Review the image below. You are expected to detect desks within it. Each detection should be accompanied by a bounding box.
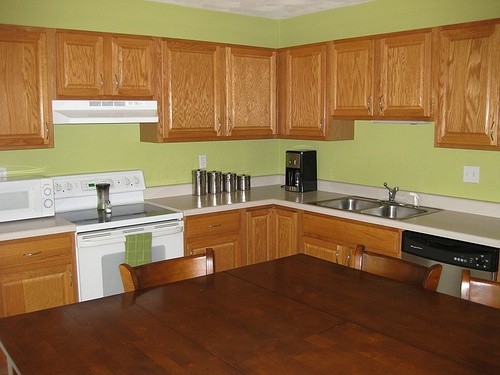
[1,253,500,375]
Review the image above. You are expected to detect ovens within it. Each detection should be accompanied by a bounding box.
[75,220,185,303]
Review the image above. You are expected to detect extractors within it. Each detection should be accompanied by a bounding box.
[51,99,159,124]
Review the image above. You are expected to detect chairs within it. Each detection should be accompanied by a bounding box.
[119,248,217,292]
[459,269,499,308]
[353,243,442,291]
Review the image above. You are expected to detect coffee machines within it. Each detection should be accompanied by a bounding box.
[285,149,317,192]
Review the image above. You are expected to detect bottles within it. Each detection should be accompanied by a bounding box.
[96,183,111,210]
[105,200,112,213]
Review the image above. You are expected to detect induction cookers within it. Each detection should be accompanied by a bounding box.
[49,170,184,233]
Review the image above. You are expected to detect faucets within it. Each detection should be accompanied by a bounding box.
[383,182,406,206]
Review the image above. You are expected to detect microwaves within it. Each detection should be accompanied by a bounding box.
[0,174,56,223]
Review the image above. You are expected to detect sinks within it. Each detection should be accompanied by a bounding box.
[304,196,381,210]
[360,206,441,220]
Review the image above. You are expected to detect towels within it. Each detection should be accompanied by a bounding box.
[124,232,152,267]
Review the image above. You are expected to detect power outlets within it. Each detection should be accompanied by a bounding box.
[463,165,480,183]
[0,168,7,179]
[199,155,206,169]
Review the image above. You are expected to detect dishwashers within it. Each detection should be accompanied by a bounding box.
[400,229,500,300]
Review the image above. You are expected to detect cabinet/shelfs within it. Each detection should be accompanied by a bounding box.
[277,43,354,141]
[184,210,243,273]
[1,22,57,151]
[54,30,154,101]
[296,212,403,273]
[330,29,435,122]
[433,17,500,150]
[141,40,278,143]
[0,233,78,317]
[244,206,274,265]
[275,204,298,259]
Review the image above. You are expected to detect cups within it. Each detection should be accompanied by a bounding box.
[208,170,222,194]
[238,174,250,190]
[222,172,237,192]
[192,169,206,196]
